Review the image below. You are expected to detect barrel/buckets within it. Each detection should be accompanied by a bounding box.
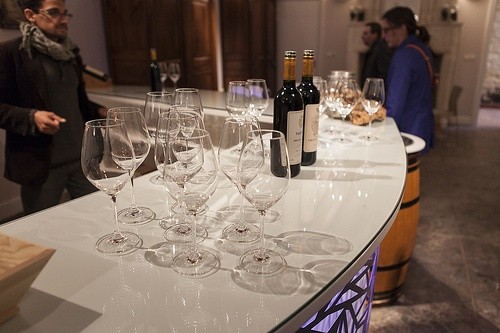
[365,151,424,298]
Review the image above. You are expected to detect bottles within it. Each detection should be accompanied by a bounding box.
[149,47,162,92]
[270,50,304,177]
[82,65,109,82]
[297,49,320,167]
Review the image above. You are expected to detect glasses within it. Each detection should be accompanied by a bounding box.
[383,24,401,34]
[30,7,73,20]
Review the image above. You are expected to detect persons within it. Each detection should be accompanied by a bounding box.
[379,6,435,157]
[360,22,396,92]
[0,0,109,215]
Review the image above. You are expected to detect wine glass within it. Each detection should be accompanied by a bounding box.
[313,71,360,148]
[158,62,168,91]
[360,77,385,142]
[80,78,291,278]
[168,63,181,90]
